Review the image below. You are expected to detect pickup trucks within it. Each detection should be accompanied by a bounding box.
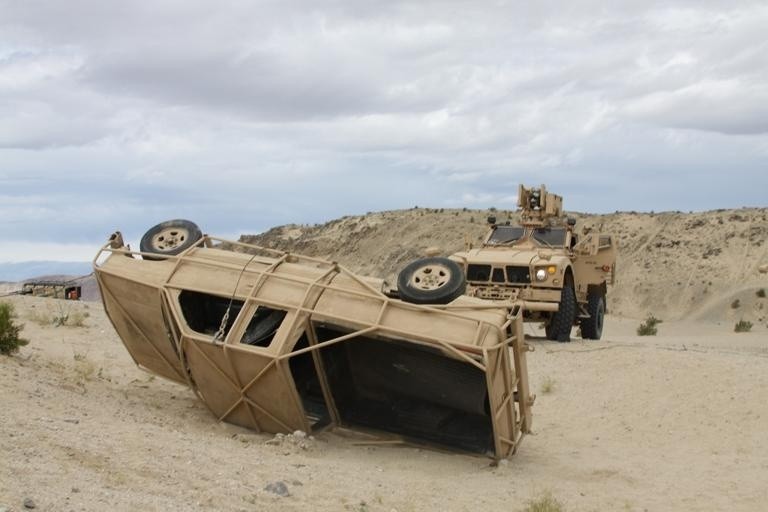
[91,216,537,469]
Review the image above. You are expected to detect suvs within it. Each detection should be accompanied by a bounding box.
[12,281,82,300]
[426,184,620,342]
